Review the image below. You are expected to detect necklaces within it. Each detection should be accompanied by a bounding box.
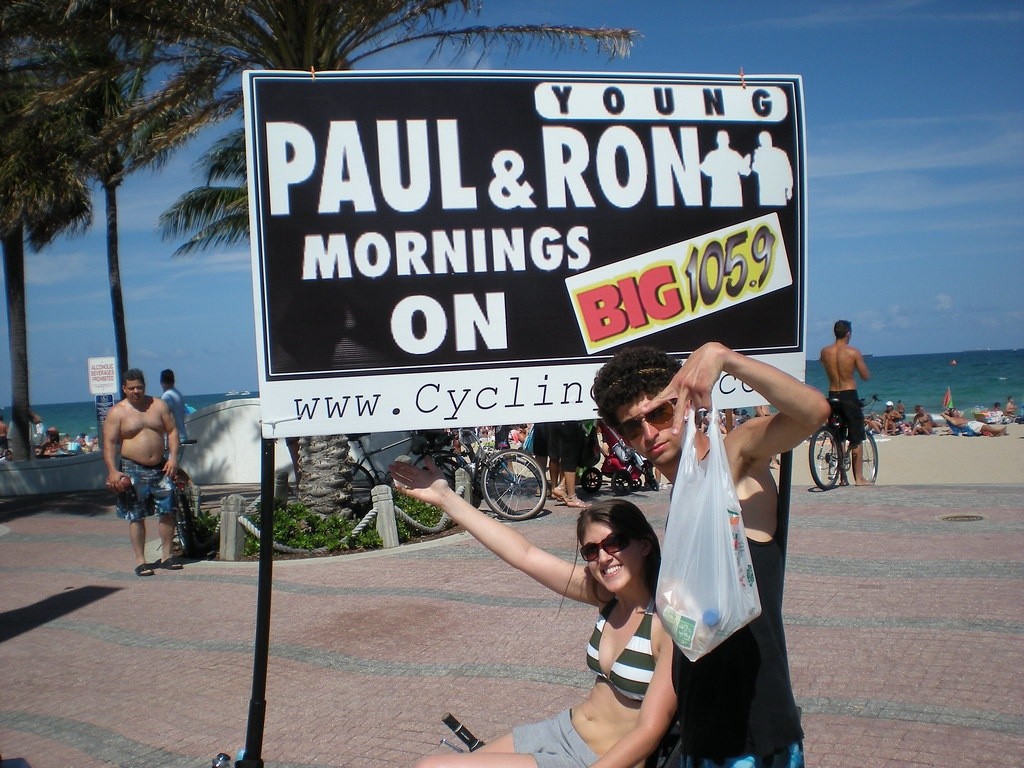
[702,449,710,459]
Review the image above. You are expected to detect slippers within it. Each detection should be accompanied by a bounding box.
[134,563,154,576]
[157,558,183,570]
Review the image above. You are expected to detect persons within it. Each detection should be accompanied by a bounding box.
[103,369,183,576]
[991,397,1017,423]
[479,420,602,508]
[755,406,779,469]
[388,456,678,768]
[911,405,941,435]
[0,416,8,455]
[697,405,751,433]
[863,400,904,436]
[160,369,187,443]
[593,341,832,768]
[941,408,1008,437]
[655,467,668,489]
[7,408,98,458]
[821,320,873,487]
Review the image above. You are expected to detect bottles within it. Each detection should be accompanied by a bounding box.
[470,457,476,479]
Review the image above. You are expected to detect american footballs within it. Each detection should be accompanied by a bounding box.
[106,476,132,492]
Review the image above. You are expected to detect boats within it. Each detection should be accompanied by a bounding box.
[0,398,439,497]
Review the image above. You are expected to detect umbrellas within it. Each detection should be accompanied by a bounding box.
[944,387,953,409]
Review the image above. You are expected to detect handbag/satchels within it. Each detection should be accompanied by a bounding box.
[655,401,762,663]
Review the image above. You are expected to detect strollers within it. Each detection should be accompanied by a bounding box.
[582,419,660,496]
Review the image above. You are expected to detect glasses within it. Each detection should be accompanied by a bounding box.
[615,399,677,441]
[579,531,630,561]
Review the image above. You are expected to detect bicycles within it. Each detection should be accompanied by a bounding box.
[342,428,548,522]
[808,392,879,491]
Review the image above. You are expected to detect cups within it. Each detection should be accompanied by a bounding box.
[107,476,131,492]
